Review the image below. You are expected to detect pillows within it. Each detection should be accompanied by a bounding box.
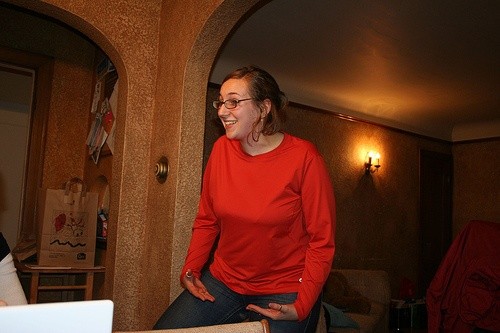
[325,305,358,328]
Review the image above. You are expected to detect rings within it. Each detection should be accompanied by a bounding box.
[279,306,282,311]
[185,272,191,278]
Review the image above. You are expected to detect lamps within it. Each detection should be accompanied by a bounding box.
[364,150,381,176]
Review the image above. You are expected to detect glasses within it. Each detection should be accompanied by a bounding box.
[212,96,256,110]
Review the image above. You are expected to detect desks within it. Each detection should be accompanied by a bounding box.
[20,263,106,304]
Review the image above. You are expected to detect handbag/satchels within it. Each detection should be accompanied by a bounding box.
[37,179,99,268]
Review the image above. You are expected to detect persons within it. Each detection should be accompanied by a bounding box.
[0,232,28,306]
[153,65,336,333]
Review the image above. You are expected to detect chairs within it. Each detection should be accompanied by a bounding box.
[320,267,391,333]
